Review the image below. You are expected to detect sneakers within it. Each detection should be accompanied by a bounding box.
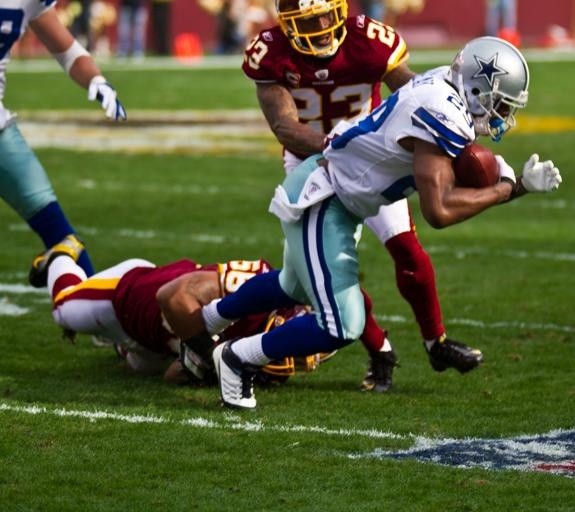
[423,334,483,374]
[361,351,397,394]
[160,310,209,386]
[210,338,262,411]
[28,233,86,291]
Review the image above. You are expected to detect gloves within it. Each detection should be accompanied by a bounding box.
[87,75,126,122]
[494,153,517,198]
[521,153,562,194]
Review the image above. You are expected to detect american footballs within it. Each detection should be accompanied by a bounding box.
[454,145,500,188]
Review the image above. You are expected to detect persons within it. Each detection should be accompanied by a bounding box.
[241,2,485,395]
[65,1,172,58]
[200,34,567,413]
[27,220,372,390]
[360,1,384,22]
[487,0,517,40]
[0,1,130,284]
[218,0,248,55]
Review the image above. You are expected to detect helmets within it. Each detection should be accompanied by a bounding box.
[444,35,531,114]
[274,0,348,60]
[274,303,338,369]
